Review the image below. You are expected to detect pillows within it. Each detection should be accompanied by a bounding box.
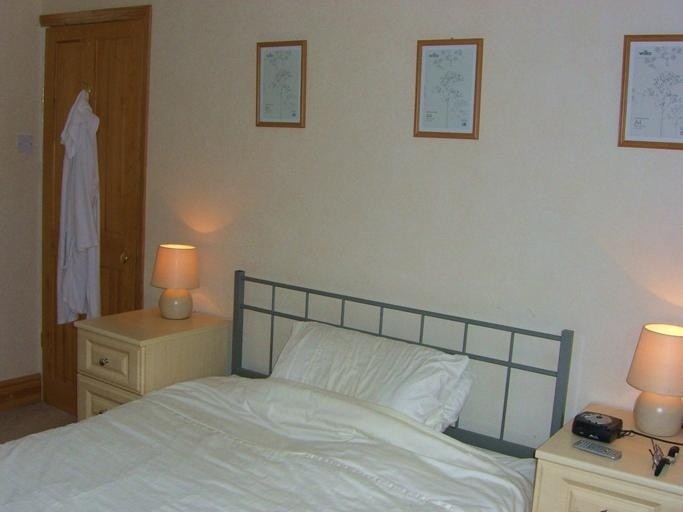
[270,319,469,425]
[425,375,473,433]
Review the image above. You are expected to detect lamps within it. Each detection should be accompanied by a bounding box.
[626,323,683,437]
[150,243,199,320]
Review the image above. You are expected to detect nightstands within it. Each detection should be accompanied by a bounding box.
[531,402,683,512]
[73,308,233,421]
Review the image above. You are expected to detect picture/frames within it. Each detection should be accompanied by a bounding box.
[618,34,683,150]
[255,40,308,129]
[413,37,484,141]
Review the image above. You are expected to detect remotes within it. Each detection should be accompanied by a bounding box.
[573,439,622,461]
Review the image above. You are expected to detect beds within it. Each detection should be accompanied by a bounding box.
[0,270,575,512]
